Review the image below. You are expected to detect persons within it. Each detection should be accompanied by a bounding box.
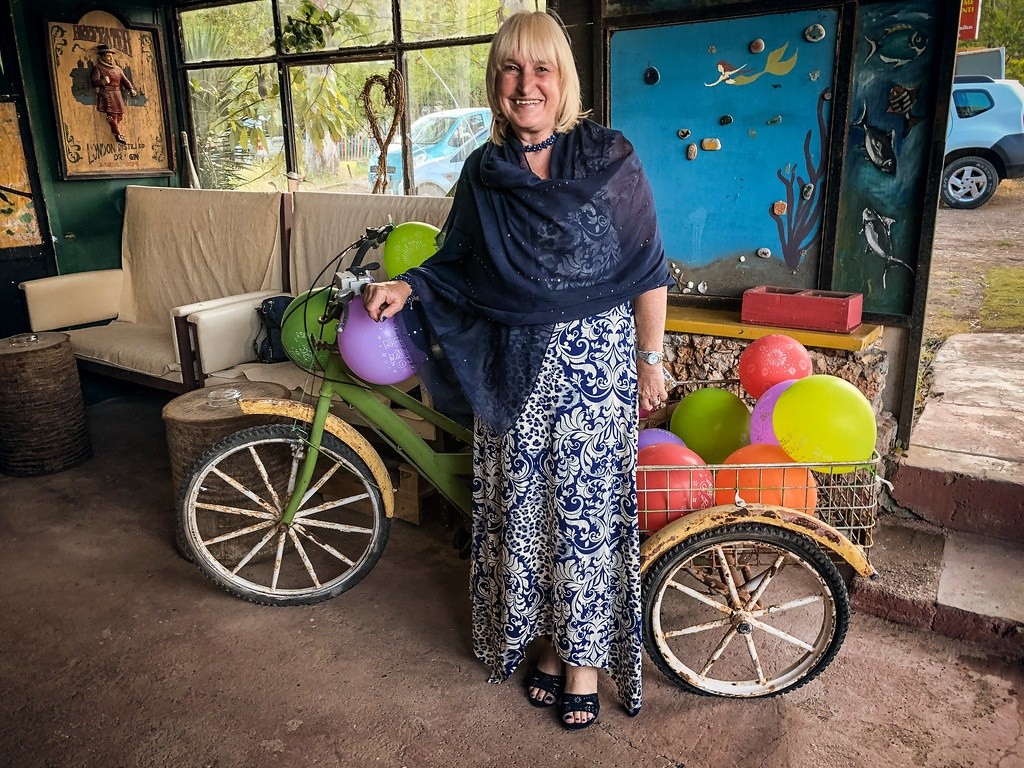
[92,45,137,144]
[361,12,677,730]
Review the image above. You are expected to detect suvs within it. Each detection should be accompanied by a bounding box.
[940,75,1024,210]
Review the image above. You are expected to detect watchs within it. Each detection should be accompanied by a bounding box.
[637,350,664,365]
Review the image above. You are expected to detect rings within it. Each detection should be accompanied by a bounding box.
[651,399,658,404]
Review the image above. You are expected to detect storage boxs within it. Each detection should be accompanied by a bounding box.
[742,284,863,332]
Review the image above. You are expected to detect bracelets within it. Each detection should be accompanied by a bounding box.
[391,275,417,304]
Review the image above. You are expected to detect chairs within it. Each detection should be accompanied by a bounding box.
[17,184,455,454]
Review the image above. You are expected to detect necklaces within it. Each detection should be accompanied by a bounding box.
[524,133,559,152]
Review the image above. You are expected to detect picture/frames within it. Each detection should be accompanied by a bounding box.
[0,95,53,259]
[593,0,860,310]
[44,20,180,181]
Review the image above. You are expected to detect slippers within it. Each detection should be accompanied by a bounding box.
[561,692,600,729]
[527,655,563,707]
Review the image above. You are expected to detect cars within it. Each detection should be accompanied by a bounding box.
[227,113,287,165]
[366,107,493,197]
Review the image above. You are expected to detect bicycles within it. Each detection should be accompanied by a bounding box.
[175,213,882,701]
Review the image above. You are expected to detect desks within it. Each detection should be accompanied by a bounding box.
[0,334,93,474]
[163,379,315,565]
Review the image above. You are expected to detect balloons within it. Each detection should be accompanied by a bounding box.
[638,427,686,449]
[636,443,715,535]
[749,379,796,445]
[715,445,816,516]
[670,388,752,475]
[280,287,343,372]
[384,222,447,281]
[773,374,876,474]
[337,295,423,385]
[739,334,813,401]
